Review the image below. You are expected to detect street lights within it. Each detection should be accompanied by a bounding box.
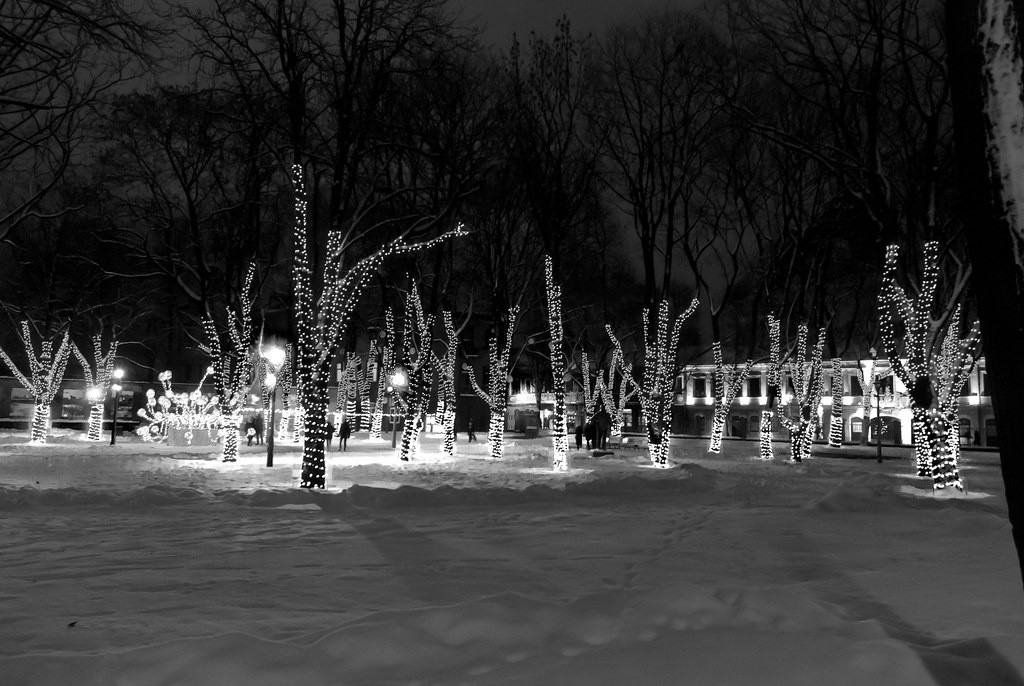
[391,373,405,450]
[872,380,883,464]
[109,369,125,446]
[266,349,286,469]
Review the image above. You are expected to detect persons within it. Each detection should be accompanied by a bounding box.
[338,419,350,452]
[324,422,336,451]
[574,423,591,450]
[245,413,264,445]
[467,417,477,442]
[590,404,613,449]
